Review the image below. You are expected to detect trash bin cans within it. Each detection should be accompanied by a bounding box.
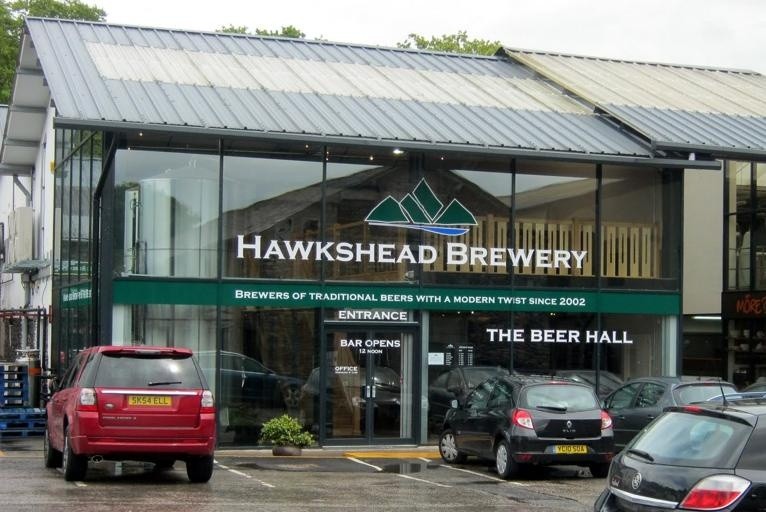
[16,349,41,408]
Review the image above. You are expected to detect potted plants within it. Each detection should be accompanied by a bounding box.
[256,411,315,457]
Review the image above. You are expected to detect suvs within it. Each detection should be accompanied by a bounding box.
[41,345,216,483]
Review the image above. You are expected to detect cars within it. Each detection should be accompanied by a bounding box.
[427,366,625,442]
[193,350,306,410]
[591,397,765,511]
[438,375,616,479]
[602,374,738,446]
[297,365,402,439]
